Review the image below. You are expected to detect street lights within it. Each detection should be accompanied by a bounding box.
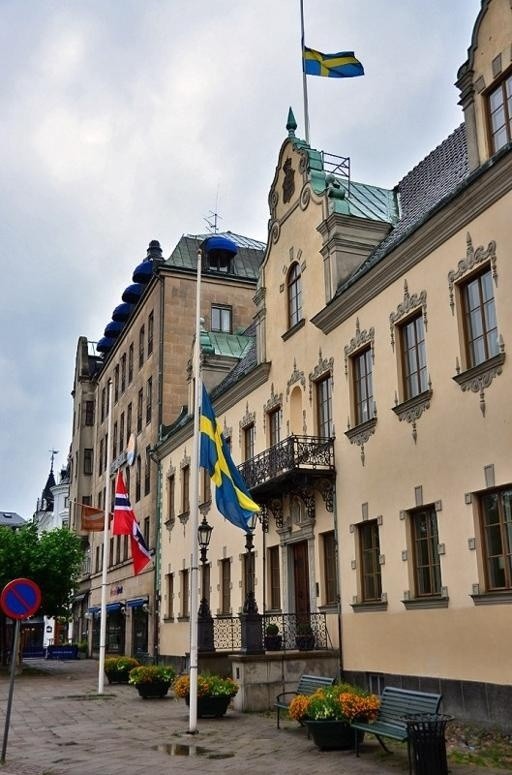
[198,515,215,653]
[238,513,266,655]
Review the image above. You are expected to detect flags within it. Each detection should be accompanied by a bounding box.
[301,45,366,78]
[81,506,114,532]
[113,470,152,577]
[197,382,261,534]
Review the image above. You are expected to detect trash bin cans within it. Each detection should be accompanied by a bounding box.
[400,712,455,775]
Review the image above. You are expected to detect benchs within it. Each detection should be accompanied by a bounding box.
[349,686,444,775]
[272,674,336,739]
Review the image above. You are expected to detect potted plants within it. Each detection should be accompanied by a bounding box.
[288,682,380,750]
[97,653,140,685]
[172,672,240,717]
[128,664,176,697]
